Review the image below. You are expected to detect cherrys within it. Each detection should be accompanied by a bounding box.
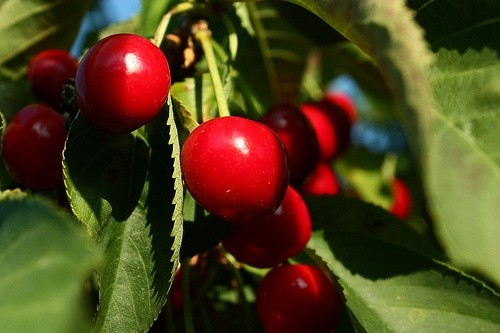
[4,33,412,332]
